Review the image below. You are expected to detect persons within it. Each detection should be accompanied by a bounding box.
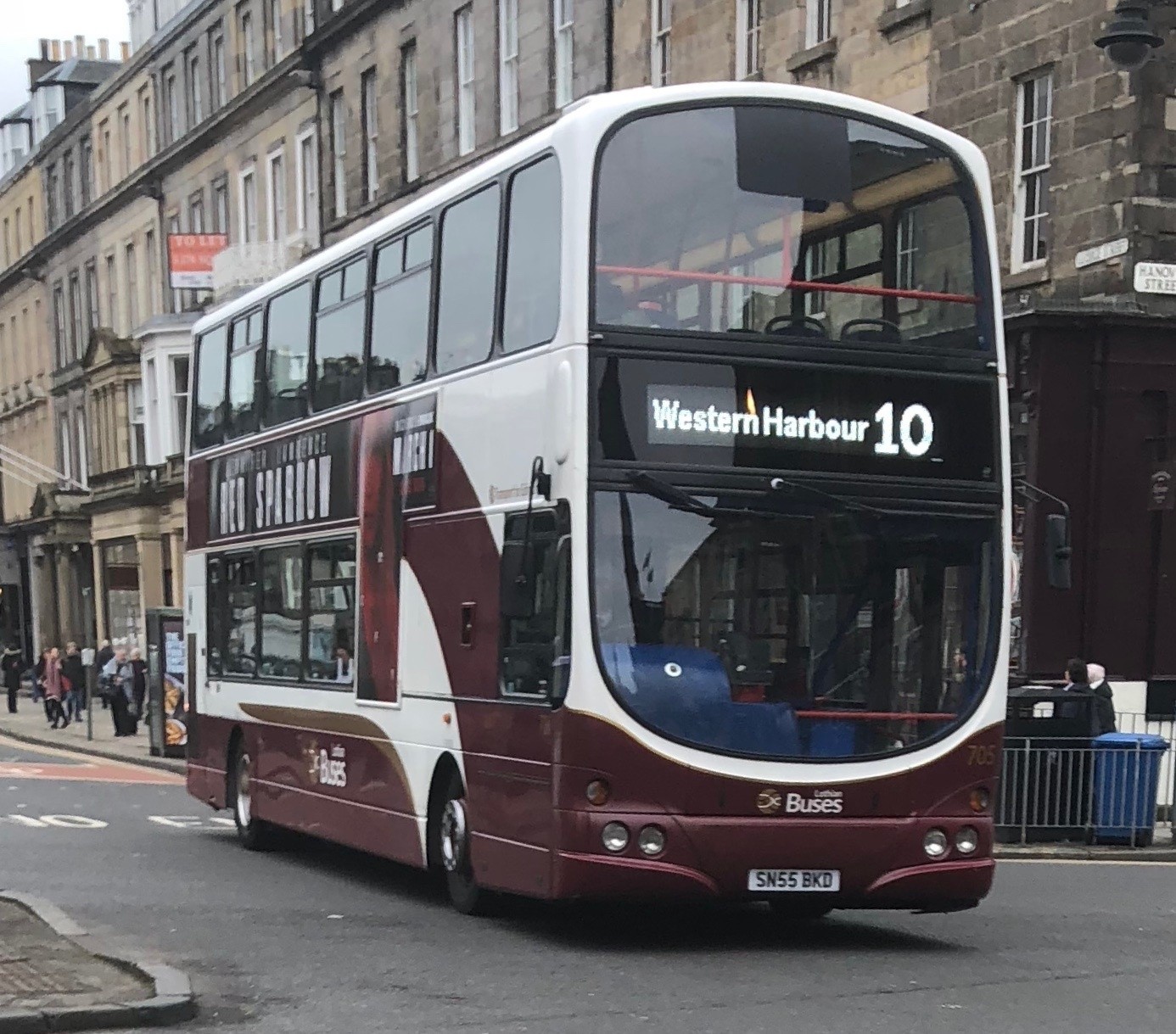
[1,639,147,737]
[954,641,967,681]
[1053,658,1117,735]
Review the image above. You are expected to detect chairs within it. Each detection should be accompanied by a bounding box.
[218,649,337,682]
[192,358,394,443]
[760,314,901,342]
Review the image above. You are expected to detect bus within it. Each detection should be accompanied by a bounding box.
[186,80,1074,931]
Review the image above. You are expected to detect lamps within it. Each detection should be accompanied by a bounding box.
[286,67,325,91]
[22,267,46,286]
[135,181,163,200]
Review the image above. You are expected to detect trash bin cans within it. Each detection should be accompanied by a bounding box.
[1092,732,1167,848]
[996,684,1096,846]
